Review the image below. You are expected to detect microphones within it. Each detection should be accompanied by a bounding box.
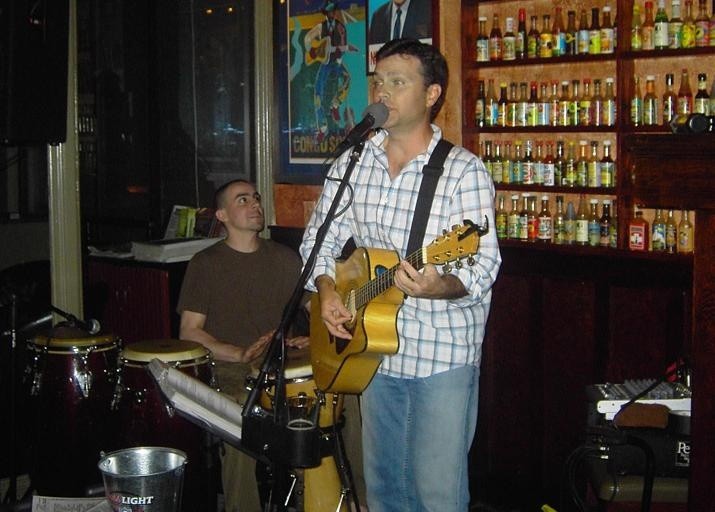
[41,302,101,335]
[331,103,389,158]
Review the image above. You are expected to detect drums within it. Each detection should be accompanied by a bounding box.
[22,324,122,497]
[250,342,348,512]
[114,338,223,511]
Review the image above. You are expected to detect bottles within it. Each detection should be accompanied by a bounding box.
[569,80,581,125]
[474,82,486,126]
[587,141,600,188]
[502,17,513,60]
[513,140,522,185]
[515,9,527,60]
[600,200,610,247]
[580,11,590,54]
[489,12,500,60]
[539,16,551,57]
[667,113,710,133]
[554,196,565,244]
[554,138,567,187]
[581,80,592,125]
[669,2,682,48]
[533,141,544,185]
[678,66,693,114]
[520,193,529,241]
[710,1,715,47]
[576,194,588,246]
[663,72,677,124]
[548,81,559,125]
[507,83,516,125]
[629,211,649,251]
[503,142,513,184]
[528,196,538,242]
[602,79,617,125]
[522,140,533,185]
[477,17,487,62]
[559,82,570,125]
[631,5,641,50]
[630,75,643,124]
[486,79,499,125]
[538,196,551,243]
[666,210,678,255]
[610,199,617,247]
[538,81,550,125]
[477,140,483,162]
[695,0,709,45]
[679,210,693,254]
[483,141,492,176]
[525,15,539,58]
[681,1,695,47]
[551,9,567,53]
[493,142,503,183]
[655,1,668,49]
[600,142,613,187]
[593,78,602,126]
[576,140,588,186]
[653,209,665,252]
[641,2,655,49]
[496,194,508,238]
[614,7,618,50]
[566,140,576,187]
[600,7,613,52]
[567,12,578,54]
[508,195,520,239]
[527,82,539,125]
[517,82,528,126]
[588,199,600,246]
[543,140,555,186]
[565,200,576,245]
[694,72,710,115]
[498,84,508,125]
[588,8,600,52]
[644,74,658,125]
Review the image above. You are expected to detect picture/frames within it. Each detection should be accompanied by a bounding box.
[272,0,368,184]
[366,0,440,75]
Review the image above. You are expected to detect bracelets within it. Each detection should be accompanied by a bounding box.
[240,348,248,365]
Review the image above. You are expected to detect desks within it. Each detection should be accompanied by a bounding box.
[82,238,205,341]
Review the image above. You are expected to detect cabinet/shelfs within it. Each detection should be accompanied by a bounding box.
[460,1,715,457]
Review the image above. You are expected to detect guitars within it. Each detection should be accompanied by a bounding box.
[310,215,488,393]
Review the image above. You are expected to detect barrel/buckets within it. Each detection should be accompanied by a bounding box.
[97,446,190,512]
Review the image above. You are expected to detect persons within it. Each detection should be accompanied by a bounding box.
[175,180,310,512]
[370,0,433,42]
[298,37,502,512]
